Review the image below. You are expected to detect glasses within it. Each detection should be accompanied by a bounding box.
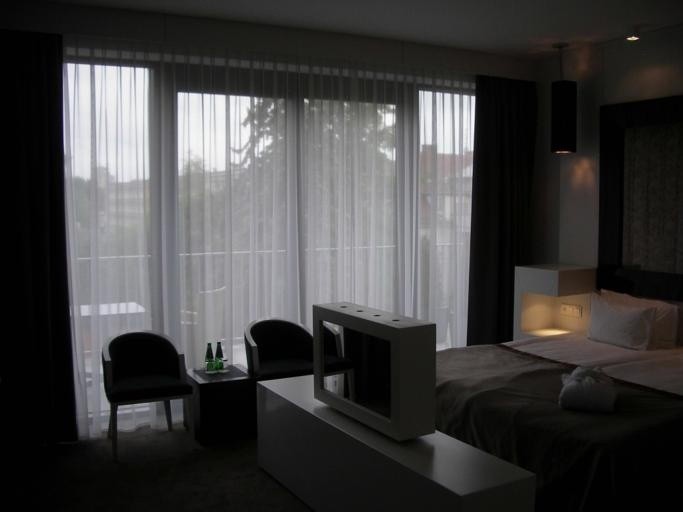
[547,40,580,156]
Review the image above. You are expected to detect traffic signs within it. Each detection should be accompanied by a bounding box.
[558,303,582,318]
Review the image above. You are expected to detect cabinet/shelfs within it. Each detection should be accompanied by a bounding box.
[215,341,224,370]
[204,342,215,372]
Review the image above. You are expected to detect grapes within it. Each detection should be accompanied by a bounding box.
[511,262,596,343]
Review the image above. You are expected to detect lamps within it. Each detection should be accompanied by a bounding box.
[244,318,314,379]
[99,331,194,440]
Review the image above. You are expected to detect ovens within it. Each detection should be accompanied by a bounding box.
[433,272,683,511]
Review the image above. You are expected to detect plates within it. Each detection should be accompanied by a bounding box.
[256,375,536,511]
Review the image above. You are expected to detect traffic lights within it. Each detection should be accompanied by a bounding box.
[588,291,654,352]
[632,296,680,349]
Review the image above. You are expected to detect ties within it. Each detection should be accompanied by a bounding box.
[311,302,438,444]
